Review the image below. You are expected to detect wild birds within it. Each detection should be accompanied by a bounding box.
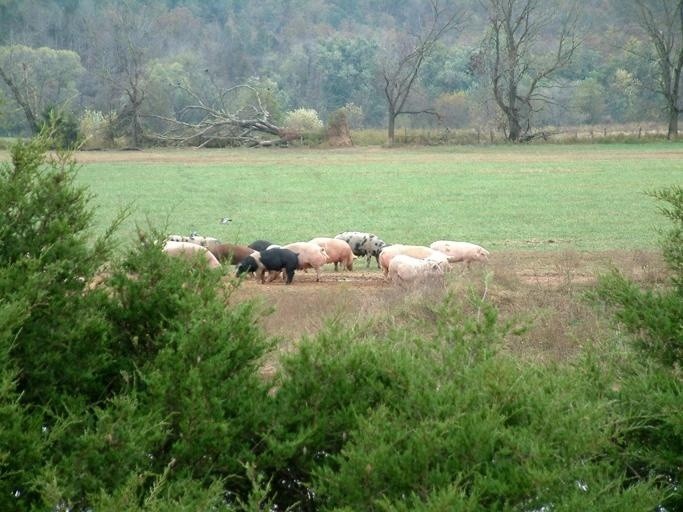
[220,217,232,224]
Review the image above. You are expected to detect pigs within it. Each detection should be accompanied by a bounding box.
[150,232,392,286]
[378,239,487,285]
